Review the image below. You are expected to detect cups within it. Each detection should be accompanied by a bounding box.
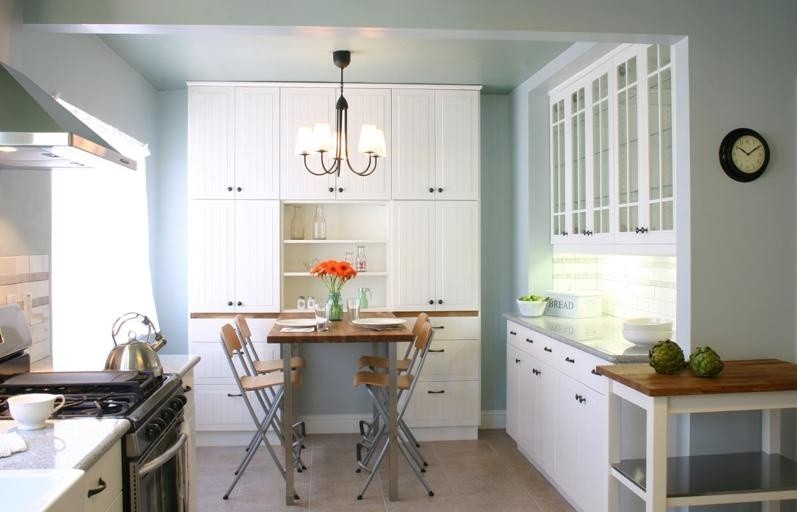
[6,393,65,430]
[346,298,361,322]
[315,304,329,332]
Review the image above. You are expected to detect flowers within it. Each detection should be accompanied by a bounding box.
[309,259,358,312]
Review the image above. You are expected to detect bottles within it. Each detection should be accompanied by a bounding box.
[290,206,305,240]
[356,245,367,272]
[312,204,326,240]
[345,252,354,266]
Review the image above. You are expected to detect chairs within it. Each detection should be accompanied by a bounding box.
[233,314,307,470]
[220,324,302,500]
[354,322,434,500]
[357,312,430,466]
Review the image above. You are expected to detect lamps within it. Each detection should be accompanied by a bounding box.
[296,50,387,176]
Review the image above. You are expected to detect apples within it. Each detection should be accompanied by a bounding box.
[519,294,544,302]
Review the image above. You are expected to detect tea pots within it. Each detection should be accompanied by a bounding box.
[104,312,167,381]
[276,318,327,328]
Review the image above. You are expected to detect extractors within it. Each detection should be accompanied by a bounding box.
[1,60,136,172]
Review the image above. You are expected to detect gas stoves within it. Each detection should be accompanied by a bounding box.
[0,373,194,458]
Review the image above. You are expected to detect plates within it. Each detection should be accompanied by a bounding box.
[352,318,406,330]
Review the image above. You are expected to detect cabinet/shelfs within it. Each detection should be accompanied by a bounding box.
[82,439,123,512]
[186,81,280,447]
[596,359,797,512]
[548,43,679,256]
[181,368,195,512]
[517,327,557,488]
[280,83,391,434]
[557,335,621,512]
[506,317,522,443]
[391,84,481,440]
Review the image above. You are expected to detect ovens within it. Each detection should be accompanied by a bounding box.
[122,410,188,512]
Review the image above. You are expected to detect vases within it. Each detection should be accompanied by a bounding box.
[326,292,344,321]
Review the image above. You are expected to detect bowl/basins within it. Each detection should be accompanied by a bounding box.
[517,299,548,318]
[624,318,673,331]
[623,331,673,347]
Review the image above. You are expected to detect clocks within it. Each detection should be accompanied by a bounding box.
[718,128,770,183]
